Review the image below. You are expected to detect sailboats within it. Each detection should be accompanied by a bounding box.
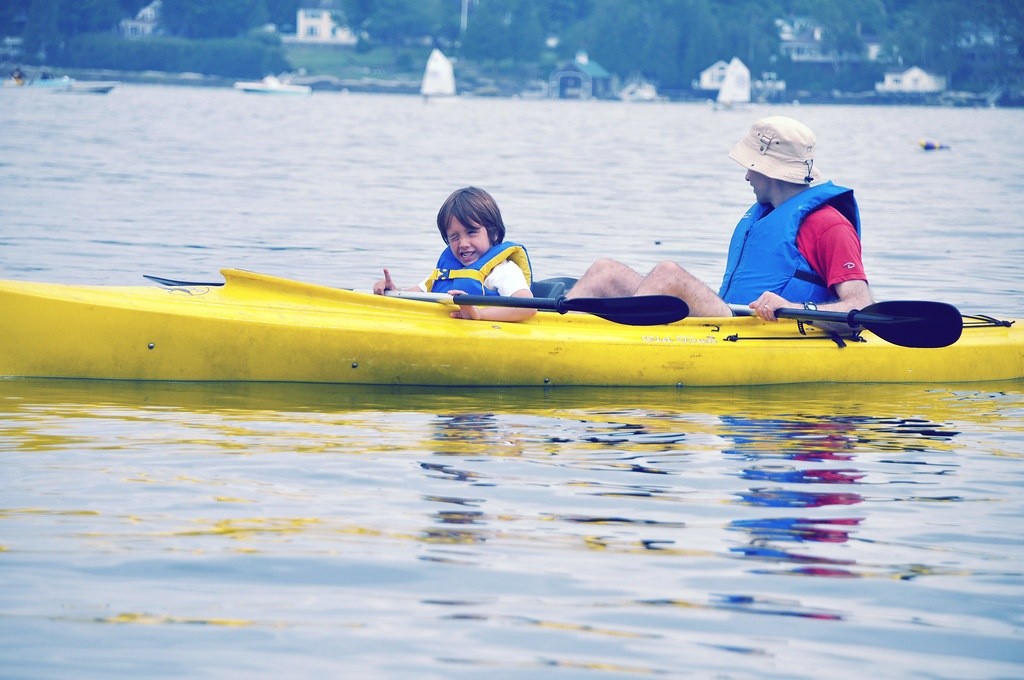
[417,47,459,100]
[712,53,752,112]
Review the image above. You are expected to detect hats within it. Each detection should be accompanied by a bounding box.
[729,116,827,184]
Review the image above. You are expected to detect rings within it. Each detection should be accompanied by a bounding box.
[765,305,771,311]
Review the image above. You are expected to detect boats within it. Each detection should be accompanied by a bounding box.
[1,266,1024,389]
[232,73,312,96]
[1,71,122,111]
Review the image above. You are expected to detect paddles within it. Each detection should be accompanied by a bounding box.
[141,272,690,327]
[535,276,963,348]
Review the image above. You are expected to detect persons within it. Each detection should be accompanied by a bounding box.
[374,187,537,321]
[566,115,876,337]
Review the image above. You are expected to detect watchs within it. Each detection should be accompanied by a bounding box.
[797,300,818,336]
